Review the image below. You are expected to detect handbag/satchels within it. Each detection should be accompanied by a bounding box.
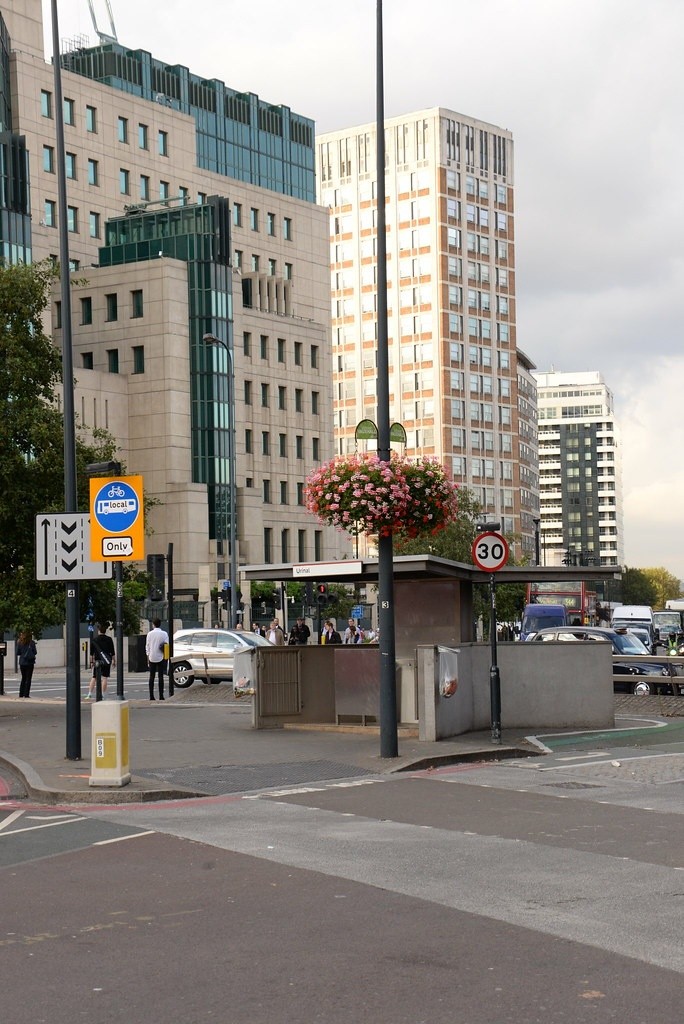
[23,644,36,662]
[99,650,112,665]
[159,641,167,652]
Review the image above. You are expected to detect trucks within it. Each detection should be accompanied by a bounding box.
[653,610,684,639]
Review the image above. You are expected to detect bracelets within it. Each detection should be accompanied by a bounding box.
[90,662,93,663]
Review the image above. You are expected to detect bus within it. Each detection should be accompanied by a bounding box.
[520,603,571,642]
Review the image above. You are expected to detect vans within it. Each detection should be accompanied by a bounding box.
[611,604,655,632]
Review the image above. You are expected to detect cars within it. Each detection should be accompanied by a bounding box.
[164,627,279,687]
[532,626,681,696]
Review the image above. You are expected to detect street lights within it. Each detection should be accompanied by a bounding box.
[202,332,238,629]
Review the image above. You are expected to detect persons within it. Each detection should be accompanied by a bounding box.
[84,624,117,700]
[16,629,37,698]
[214,616,380,644]
[145,619,169,700]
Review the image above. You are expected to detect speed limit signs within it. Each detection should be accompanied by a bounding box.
[472,531,509,572]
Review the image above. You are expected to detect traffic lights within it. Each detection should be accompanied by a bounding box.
[317,584,339,606]
